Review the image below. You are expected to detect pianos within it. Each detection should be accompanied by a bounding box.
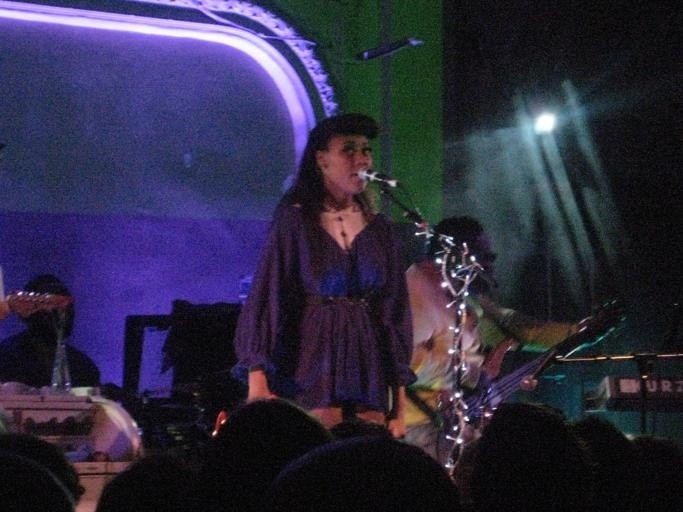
[1,380,101,432]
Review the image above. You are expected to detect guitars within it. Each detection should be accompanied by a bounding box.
[2,290,71,317]
[388,300,627,466]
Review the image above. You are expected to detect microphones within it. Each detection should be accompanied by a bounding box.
[356,167,401,189]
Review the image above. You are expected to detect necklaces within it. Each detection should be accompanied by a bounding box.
[335,208,351,249]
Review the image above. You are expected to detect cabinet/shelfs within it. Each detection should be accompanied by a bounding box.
[0,380,144,512]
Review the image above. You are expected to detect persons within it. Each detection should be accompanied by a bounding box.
[389,211,512,428]
[0,395,683,510]
[231,111,420,439]
[0,273,102,388]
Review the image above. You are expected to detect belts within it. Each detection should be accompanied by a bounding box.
[295,293,377,311]
